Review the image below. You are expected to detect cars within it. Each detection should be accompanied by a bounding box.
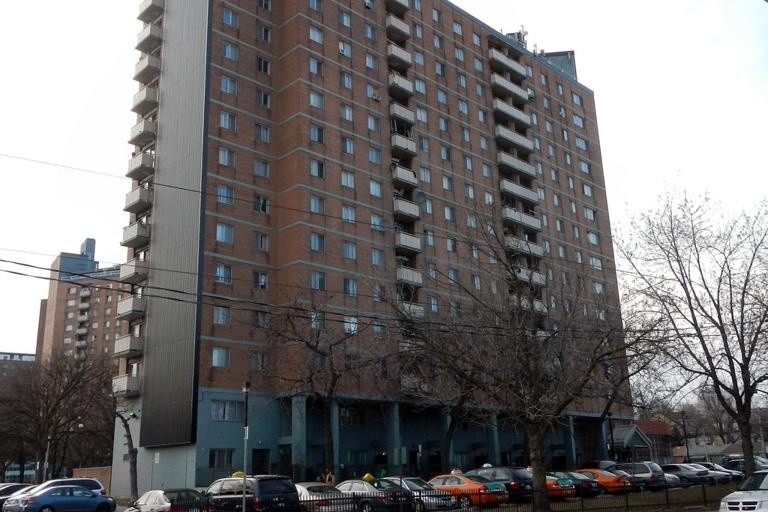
[0,475,116,510]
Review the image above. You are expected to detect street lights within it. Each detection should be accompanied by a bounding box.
[606,412,617,462]
[679,410,690,462]
[238,382,250,510]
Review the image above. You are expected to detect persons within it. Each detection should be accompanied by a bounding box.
[324,469,336,486]
